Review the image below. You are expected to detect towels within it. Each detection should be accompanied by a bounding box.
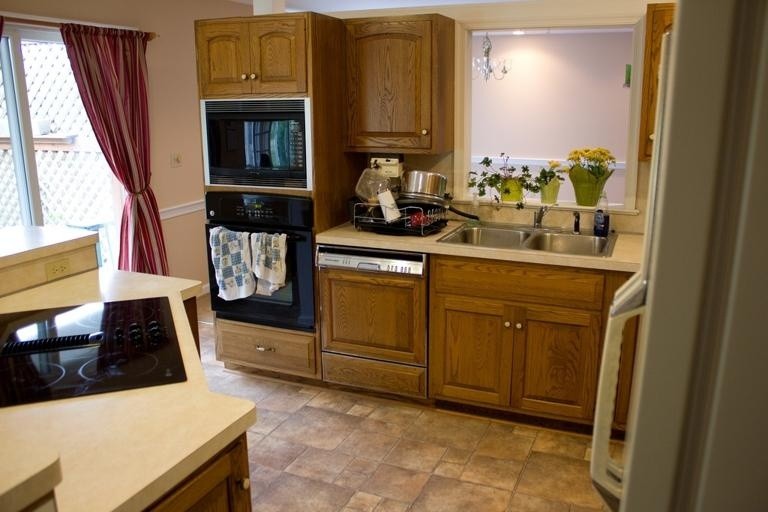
[250,228,287,295]
[208,227,255,302]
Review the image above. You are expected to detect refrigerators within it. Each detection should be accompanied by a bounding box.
[584,1,767,511]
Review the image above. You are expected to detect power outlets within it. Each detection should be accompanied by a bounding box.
[46,257,70,282]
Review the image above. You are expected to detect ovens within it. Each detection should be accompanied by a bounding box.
[203,191,315,333]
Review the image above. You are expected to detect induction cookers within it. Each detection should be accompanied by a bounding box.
[0,296,187,407]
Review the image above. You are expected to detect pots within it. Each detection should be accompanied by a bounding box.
[399,171,482,224]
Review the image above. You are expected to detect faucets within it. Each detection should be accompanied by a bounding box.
[533,203,559,229]
[572,210,580,234]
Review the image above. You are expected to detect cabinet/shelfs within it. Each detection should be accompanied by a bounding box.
[316,267,425,396]
[192,10,345,100]
[435,258,607,422]
[141,442,252,512]
[638,2,676,163]
[214,318,317,378]
[340,14,456,158]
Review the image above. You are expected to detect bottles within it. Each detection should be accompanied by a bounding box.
[593,189,610,237]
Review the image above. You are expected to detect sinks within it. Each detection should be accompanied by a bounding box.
[436,220,534,250]
[526,225,620,257]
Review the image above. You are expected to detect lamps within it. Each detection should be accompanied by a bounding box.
[470,30,508,89]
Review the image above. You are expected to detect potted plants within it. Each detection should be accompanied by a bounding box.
[470,152,539,209]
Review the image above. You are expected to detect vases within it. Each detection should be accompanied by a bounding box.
[568,165,612,207]
[538,181,562,204]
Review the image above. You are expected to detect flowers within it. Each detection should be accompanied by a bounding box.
[566,147,617,176]
[535,158,570,185]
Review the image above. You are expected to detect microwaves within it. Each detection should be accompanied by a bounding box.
[200,98,313,191]
